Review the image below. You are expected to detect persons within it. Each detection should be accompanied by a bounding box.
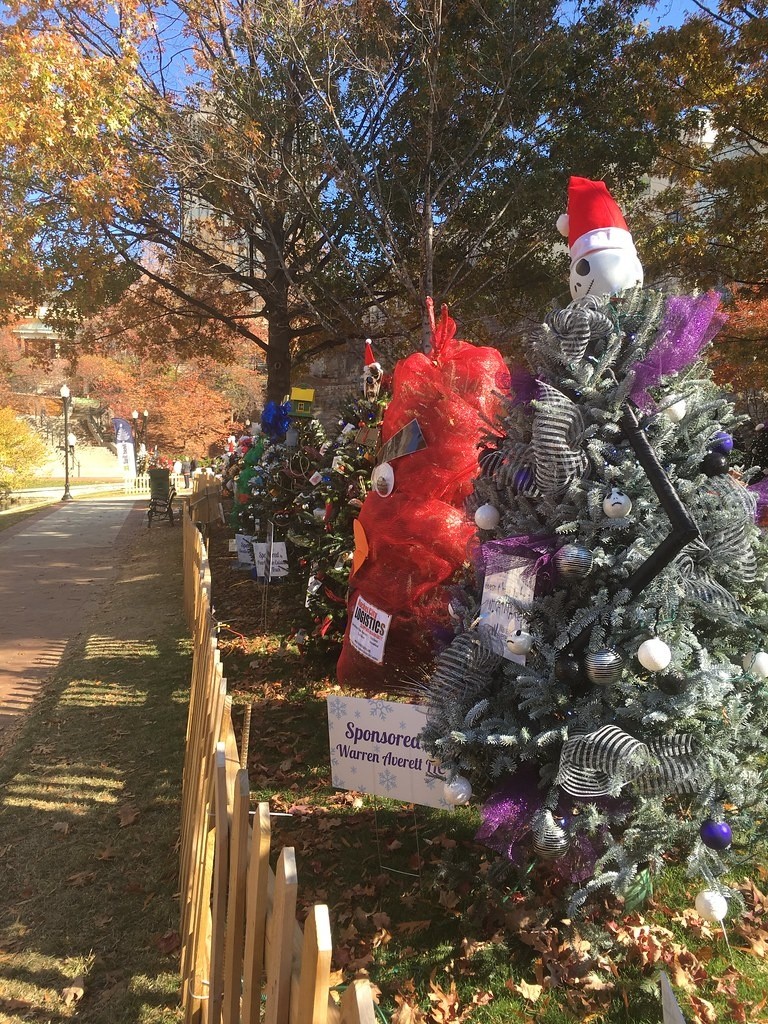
[182,458,190,488]
[190,457,197,480]
[210,457,216,472]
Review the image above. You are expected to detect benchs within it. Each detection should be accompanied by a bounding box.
[148,488,177,528]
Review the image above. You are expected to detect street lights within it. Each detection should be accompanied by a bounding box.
[132,410,138,466]
[58,384,73,501]
[143,409,148,450]
